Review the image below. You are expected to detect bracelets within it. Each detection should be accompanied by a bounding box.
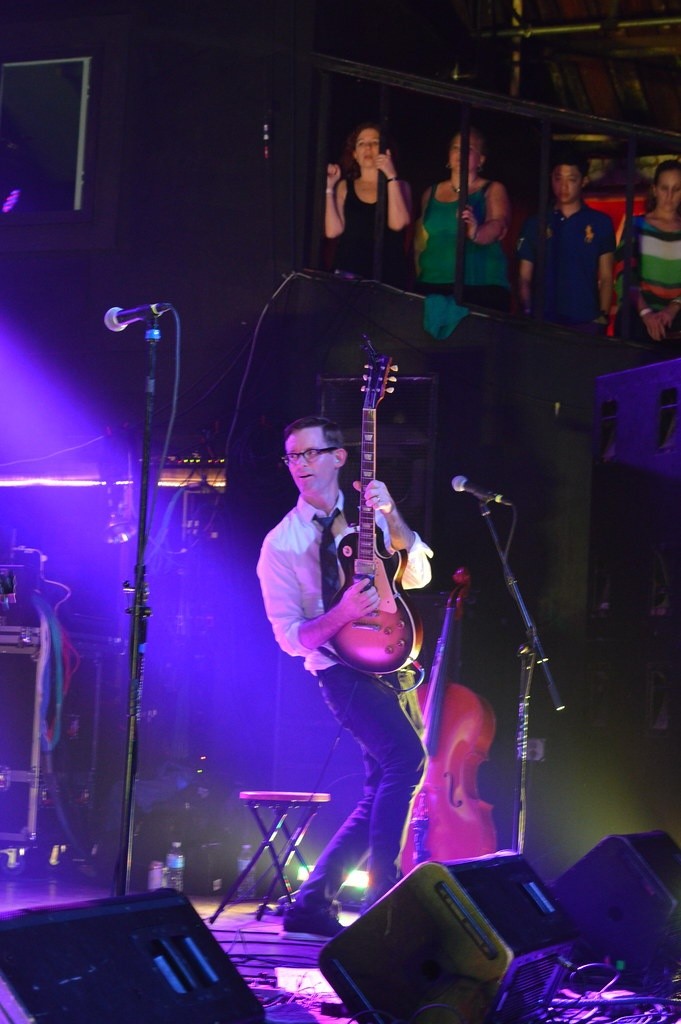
[639,308,652,316]
[600,310,609,322]
[326,189,334,193]
[386,177,400,183]
[470,232,477,241]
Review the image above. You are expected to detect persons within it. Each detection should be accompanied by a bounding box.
[517,155,616,333]
[403,128,512,311]
[258,419,434,937]
[613,159,681,354]
[324,124,415,288]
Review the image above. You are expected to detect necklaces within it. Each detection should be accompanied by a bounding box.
[450,178,478,192]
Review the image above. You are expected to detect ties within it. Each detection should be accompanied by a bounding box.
[313,507,342,614]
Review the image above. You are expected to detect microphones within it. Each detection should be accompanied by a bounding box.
[452,475,514,505]
[104,302,173,331]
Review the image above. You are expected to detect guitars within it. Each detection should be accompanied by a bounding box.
[324,351,425,676]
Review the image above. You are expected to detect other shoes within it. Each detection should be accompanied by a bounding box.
[280,905,346,941]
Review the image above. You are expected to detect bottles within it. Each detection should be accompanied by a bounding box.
[167,841,184,891]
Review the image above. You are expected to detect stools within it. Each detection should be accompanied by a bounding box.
[207,792,333,928]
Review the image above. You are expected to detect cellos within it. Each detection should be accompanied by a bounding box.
[398,560,506,880]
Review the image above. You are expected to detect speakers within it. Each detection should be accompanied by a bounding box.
[0,887,266,1024]
[548,829,681,993]
[319,849,581,1024]
[319,370,441,591]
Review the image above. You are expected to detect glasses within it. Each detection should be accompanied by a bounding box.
[281,447,338,466]
